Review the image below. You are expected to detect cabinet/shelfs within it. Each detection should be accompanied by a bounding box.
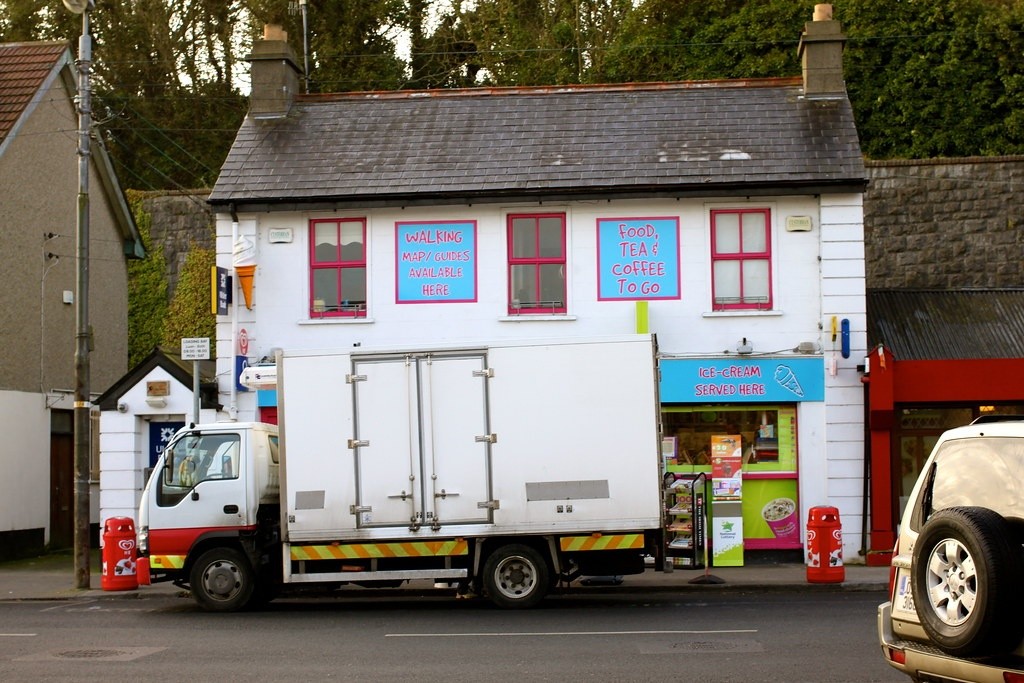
[663,471,708,568]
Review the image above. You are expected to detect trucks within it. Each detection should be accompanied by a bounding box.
[138,326,668,614]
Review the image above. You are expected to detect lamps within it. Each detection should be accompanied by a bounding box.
[736,337,753,354]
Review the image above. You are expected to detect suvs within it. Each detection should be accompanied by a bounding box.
[877,412,1023,683]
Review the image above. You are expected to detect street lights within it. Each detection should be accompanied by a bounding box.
[62,0,100,591]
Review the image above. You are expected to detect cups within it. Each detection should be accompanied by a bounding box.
[762,498,797,538]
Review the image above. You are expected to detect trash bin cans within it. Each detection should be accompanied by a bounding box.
[102,516,138,589]
[805,504,847,586]
